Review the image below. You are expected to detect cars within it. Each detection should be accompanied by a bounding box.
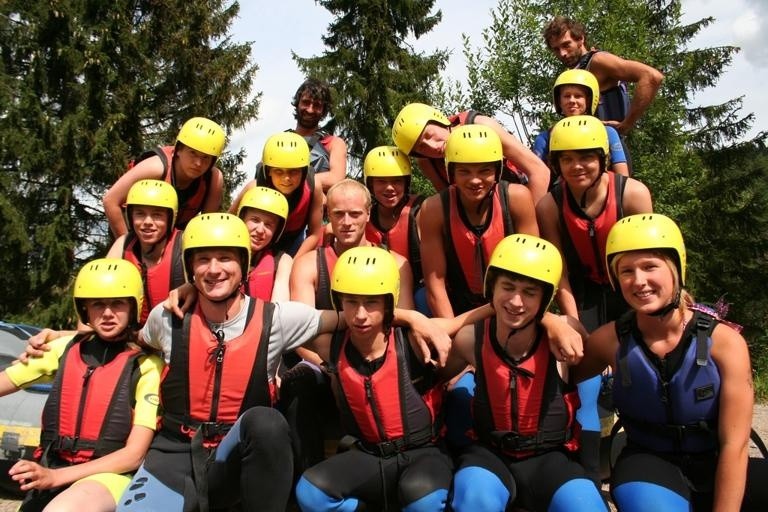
[0,320,57,392]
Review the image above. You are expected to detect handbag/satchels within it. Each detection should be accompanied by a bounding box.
[304,127,334,171]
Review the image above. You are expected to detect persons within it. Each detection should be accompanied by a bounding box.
[1,67,768,512]
[542,15,664,178]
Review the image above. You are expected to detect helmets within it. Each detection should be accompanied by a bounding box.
[181,213,253,283]
[605,214,687,291]
[237,186,290,243]
[329,246,400,316]
[173,116,226,169]
[364,69,608,191]
[262,131,310,181]
[126,179,179,232]
[72,258,144,325]
[483,235,564,318]
